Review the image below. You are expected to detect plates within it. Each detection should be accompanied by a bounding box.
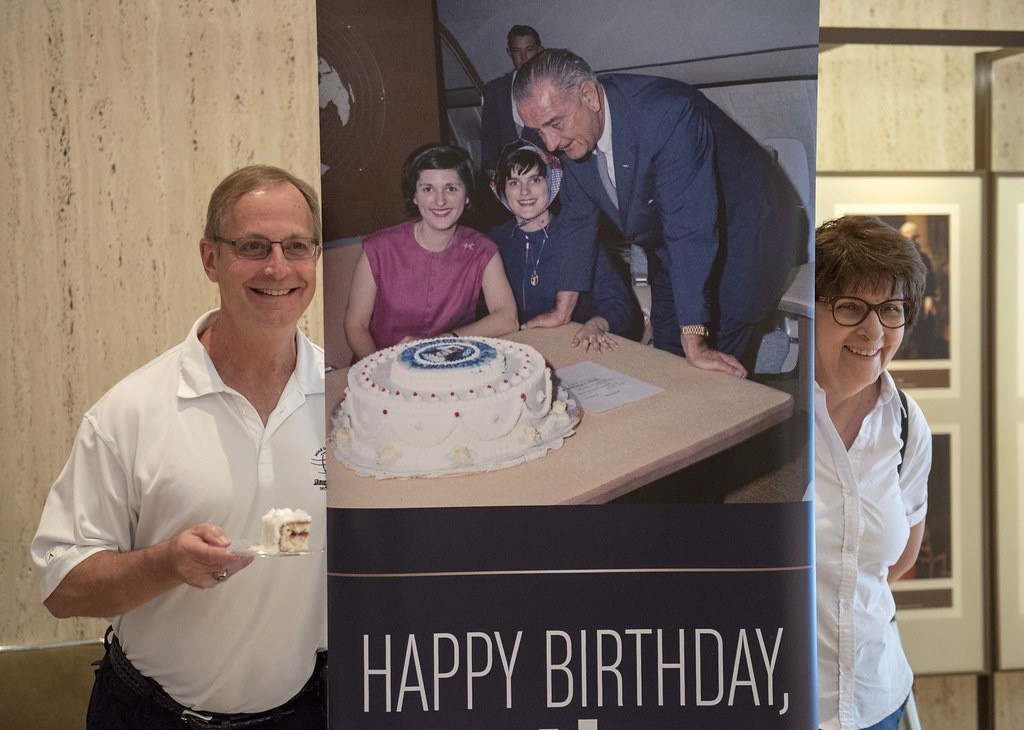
[209,537,326,556]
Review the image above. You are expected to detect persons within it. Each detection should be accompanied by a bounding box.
[511,48,810,506]
[815,215,932,730]
[490,138,645,355]
[342,145,520,367]
[480,28,561,215]
[28,165,328,729]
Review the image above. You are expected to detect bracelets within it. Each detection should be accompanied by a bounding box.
[681,326,710,336]
[448,330,459,337]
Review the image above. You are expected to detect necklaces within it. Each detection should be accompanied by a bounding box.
[841,389,863,440]
[417,223,459,251]
[524,210,551,286]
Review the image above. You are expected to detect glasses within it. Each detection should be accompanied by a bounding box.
[212,235,319,260]
[816,296,916,328]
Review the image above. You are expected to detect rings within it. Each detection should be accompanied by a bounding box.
[214,569,228,583]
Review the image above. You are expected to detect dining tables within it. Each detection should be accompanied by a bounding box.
[325,321,794,505]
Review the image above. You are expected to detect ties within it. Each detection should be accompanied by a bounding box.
[595,145,618,210]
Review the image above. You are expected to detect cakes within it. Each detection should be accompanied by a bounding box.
[257,506,313,553]
[329,336,574,472]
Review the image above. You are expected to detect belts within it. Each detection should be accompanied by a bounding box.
[109,635,324,730]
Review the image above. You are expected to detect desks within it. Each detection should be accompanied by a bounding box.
[779,267,815,413]
